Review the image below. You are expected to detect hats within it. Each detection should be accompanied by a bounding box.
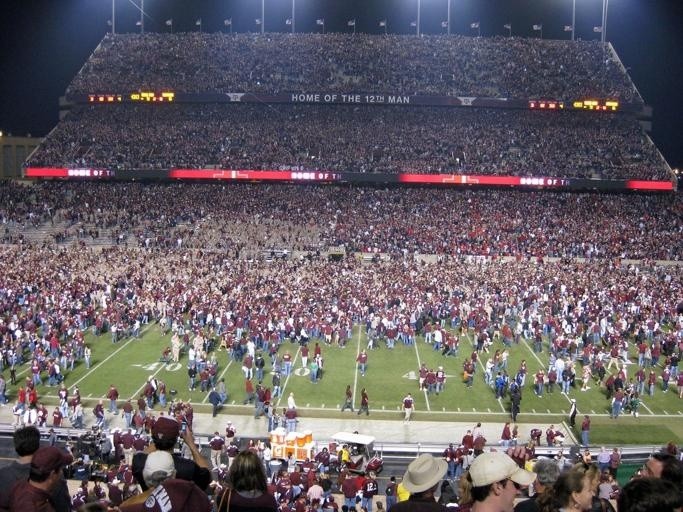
[403,454,448,493]
[468,451,537,487]
[32,447,73,472]
[143,451,176,482]
[151,418,178,438]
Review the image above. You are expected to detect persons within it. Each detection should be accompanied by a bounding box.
[1,32,683,511]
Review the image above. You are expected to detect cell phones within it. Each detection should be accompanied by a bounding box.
[181,422,187,431]
